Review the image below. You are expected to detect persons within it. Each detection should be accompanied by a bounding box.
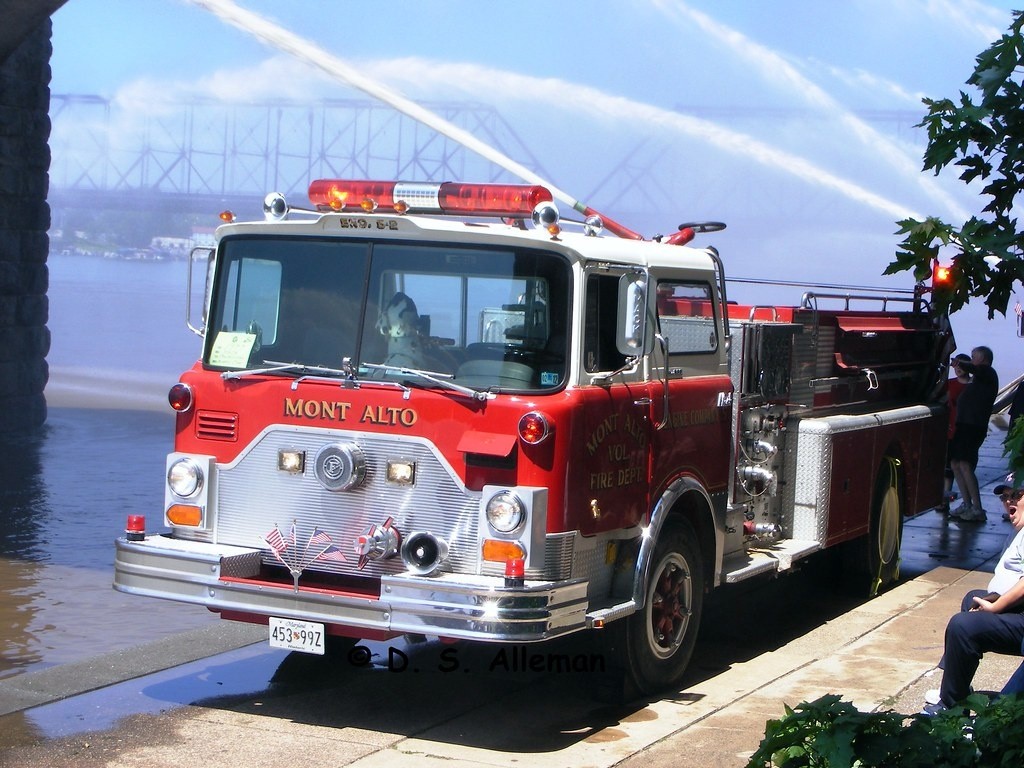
[919,468,1024,715]
[933,346,999,521]
[344,292,460,378]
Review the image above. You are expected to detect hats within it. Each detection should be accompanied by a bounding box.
[992,471,1024,495]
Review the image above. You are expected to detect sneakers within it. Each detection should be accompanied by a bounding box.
[950,502,972,517]
[961,504,987,521]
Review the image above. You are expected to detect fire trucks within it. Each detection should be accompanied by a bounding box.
[109,178,959,699]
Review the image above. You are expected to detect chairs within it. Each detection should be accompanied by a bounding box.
[459,343,554,387]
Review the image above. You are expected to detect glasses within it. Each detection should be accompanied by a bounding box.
[998,491,1024,500]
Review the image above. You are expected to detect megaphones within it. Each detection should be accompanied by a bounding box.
[401,533,449,575]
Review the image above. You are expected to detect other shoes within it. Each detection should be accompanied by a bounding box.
[923,699,945,715]
[925,687,941,704]
